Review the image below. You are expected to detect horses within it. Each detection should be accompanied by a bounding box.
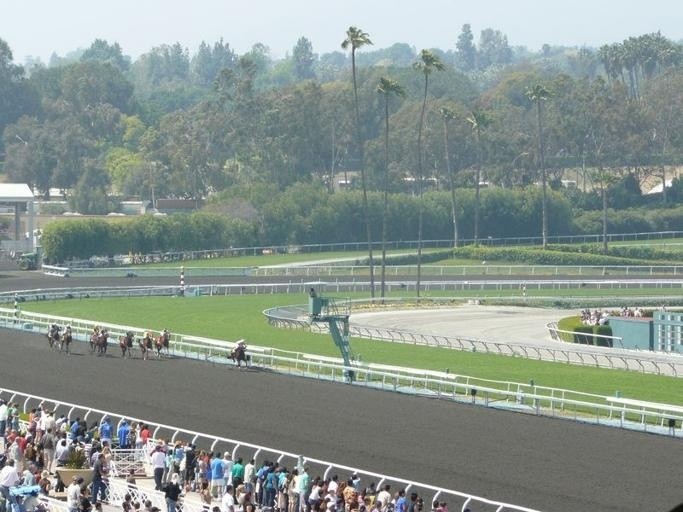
[153,332,171,358]
[137,334,153,361]
[60,328,72,355]
[231,343,249,367]
[44,326,61,352]
[88,332,109,357]
[118,331,135,358]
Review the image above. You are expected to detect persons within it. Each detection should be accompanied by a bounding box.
[231,339,246,353]
[0,392,448,512]
[46,318,171,358]
[130,250,233,264]
[309,287,318,317]
[580,305,643,326]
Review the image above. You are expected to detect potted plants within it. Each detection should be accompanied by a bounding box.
[54,447,95,487]
